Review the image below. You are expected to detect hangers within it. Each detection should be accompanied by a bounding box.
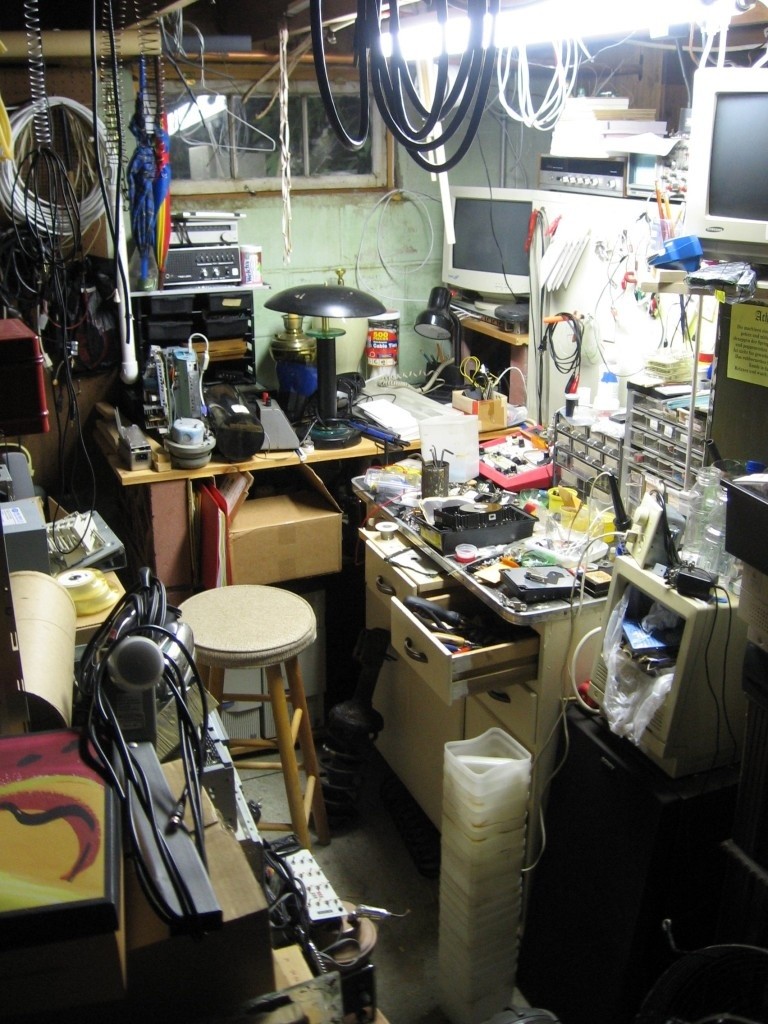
[155,18,276,152]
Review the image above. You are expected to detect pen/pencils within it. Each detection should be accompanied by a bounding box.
[430,353,437,363]
[419,349,432,363]
[654,179,665,242]
[436,344,444,363]
[662,188,674,237]
[674,200,685,224]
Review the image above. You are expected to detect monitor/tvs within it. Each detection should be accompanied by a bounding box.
[680,67,768,259]
[441,185,536,295]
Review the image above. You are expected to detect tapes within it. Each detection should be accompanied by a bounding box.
[54,567,107,599]
[75,579,121,616]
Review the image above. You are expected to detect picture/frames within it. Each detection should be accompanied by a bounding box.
[0,728,124,944]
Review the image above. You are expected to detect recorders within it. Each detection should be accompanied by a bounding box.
[536,152,630,200]
[158,244,244,287]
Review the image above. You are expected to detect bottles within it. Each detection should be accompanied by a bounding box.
[680,460,765,598]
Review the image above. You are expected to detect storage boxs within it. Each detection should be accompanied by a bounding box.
[216,590,328,745]
[451,388,508,431]
[0,861,130,1014]
[217,460,345,587]
[126,761,278,1023]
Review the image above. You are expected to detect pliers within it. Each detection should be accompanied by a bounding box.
[414,602,455,635]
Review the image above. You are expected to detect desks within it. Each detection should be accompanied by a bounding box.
[83,379,521,613]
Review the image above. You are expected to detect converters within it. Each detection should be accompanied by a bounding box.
[675,562,741,778]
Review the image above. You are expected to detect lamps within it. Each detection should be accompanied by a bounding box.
[413,286,475,404]
[263,280,386,449]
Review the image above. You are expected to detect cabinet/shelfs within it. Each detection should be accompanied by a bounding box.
[622,386,710,492]
[357,498,546,842]
[547,404,624,504]
